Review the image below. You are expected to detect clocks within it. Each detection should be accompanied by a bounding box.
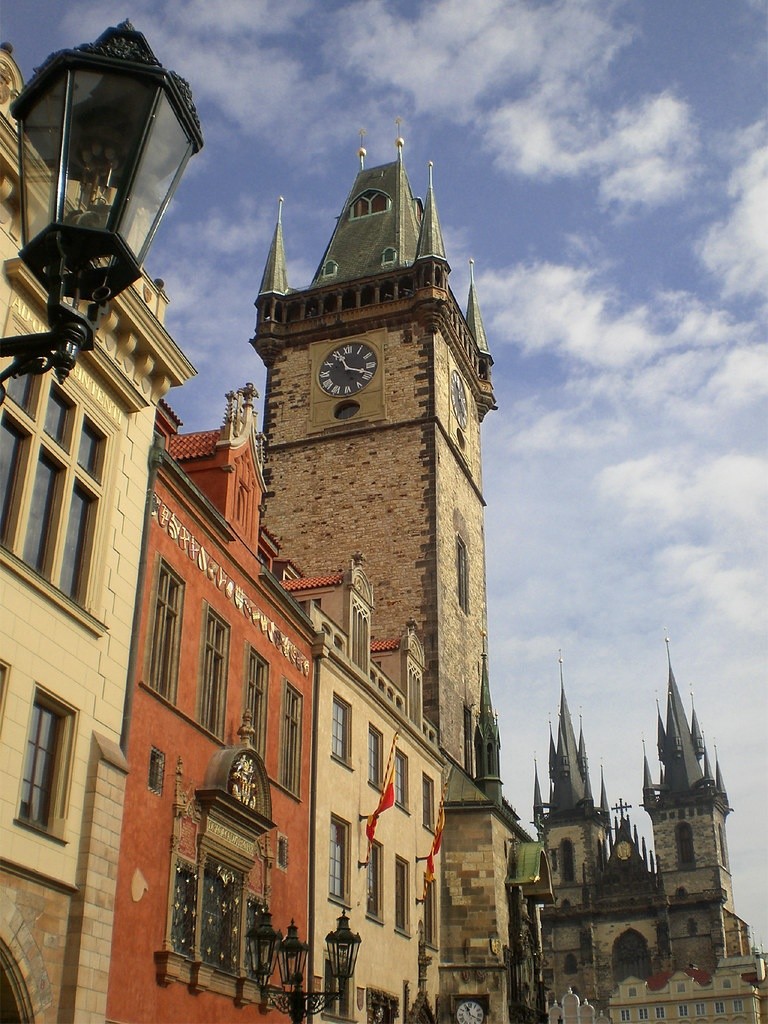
[452,370,468,428]
[319,342,378,396]
[457,1001,484,1024]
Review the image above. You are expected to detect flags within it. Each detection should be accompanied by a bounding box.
[366,733,397,865]
[422,781,448,903]
